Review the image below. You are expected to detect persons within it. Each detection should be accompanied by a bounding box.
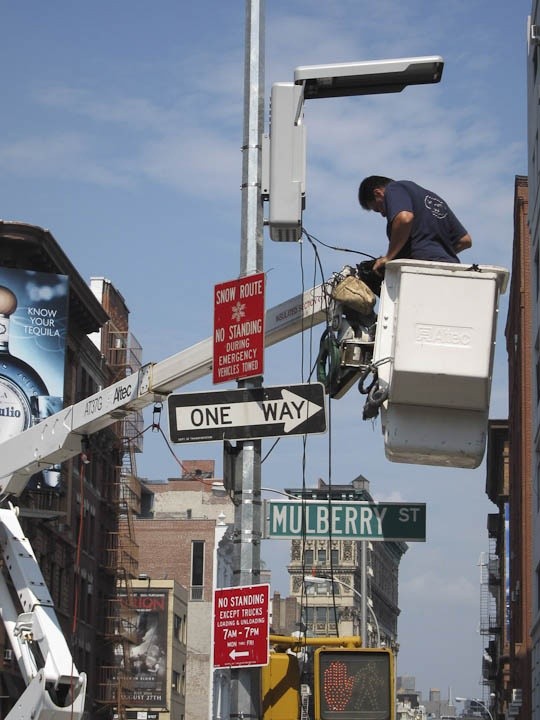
[359,175,472,271]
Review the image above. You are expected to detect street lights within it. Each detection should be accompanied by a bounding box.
[305,576,382,649]
[455,697,494,720]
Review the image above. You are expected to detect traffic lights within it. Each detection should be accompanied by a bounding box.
[314,646,396,720]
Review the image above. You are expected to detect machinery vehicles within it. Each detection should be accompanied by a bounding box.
[0,251,511,720]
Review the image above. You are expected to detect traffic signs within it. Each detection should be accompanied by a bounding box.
[213,583,269,669]
[165,382,331,444]
